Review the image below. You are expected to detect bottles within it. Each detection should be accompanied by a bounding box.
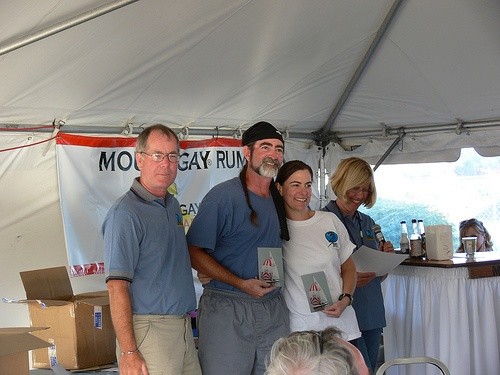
[400,221,409,254]
[418,220,427,258]
[409,219,423,258]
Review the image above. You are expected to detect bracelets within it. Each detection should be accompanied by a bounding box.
[122,350,137,355]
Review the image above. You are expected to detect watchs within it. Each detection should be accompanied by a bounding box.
[338,294,353,306]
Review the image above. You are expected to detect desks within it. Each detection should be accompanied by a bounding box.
[381,248,500,375]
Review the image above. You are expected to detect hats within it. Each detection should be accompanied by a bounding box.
[242,122,284,145]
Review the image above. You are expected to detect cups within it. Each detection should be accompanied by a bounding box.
[462,237,477,255]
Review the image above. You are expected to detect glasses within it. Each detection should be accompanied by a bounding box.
[140,152,180,163]
[352,187,369,195]
[460,218,477,226]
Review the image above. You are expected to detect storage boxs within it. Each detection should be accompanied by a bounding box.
[2,266,117,371]
[0,327,54,375]
[425,224,453,260]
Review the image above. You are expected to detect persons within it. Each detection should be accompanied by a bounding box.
[319,157,395,375]
[267,325,368,375]
[185,121,291,375]
[102,124,203,375]
[456,218,493,253]
[197,160,372,375]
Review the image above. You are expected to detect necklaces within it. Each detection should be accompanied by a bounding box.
[335,202,364,249]
